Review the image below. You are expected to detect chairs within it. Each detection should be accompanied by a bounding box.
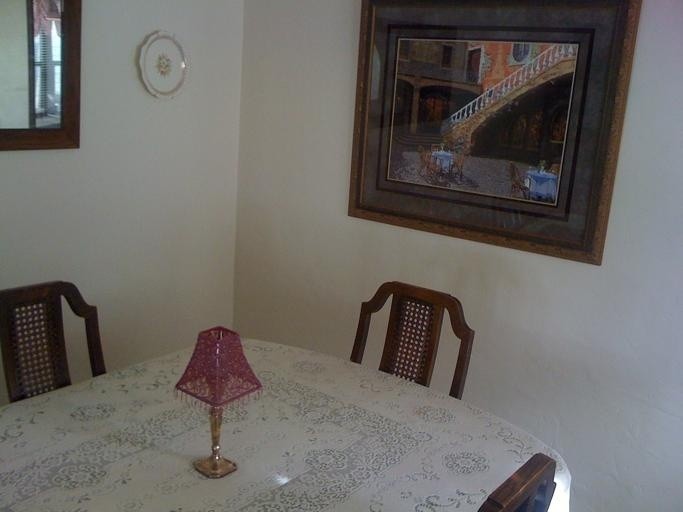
[0,283,104,412]
[350,284,473,401]
[420,140,466,183]
[510,163,532,199]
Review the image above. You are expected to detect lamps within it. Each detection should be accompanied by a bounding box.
[178,326,265,484]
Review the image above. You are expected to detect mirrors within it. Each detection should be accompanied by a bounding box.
[0,2,83,153]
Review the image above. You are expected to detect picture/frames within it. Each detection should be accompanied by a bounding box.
[347,1,646,270]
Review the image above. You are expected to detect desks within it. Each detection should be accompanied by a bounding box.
[0,341,556,510]
[525,169,556,201]
